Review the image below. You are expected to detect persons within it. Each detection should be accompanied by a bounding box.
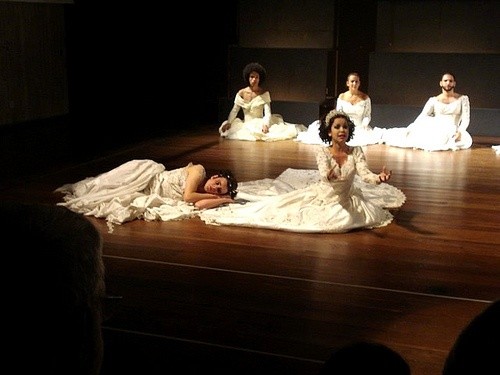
[54,158,244,234]
[296,72,383,146]
[264,112,406,233]
[219,63,308,141]
[382,72,473,151]
